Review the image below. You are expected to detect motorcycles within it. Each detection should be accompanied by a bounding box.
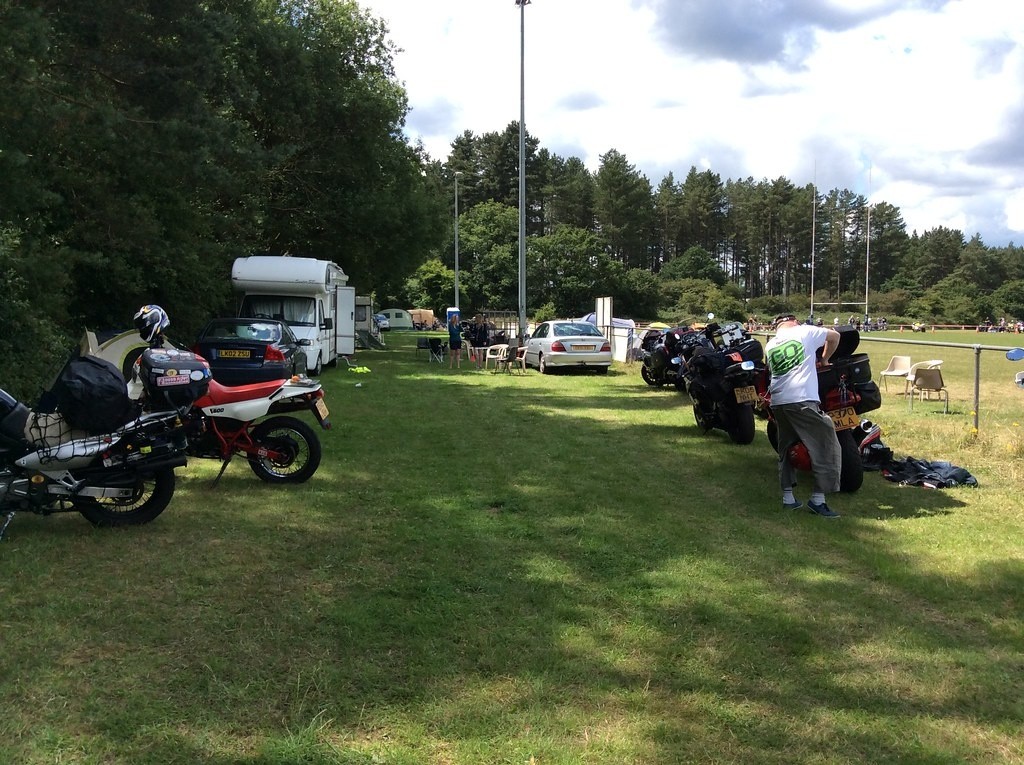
[0,347,213,528]
[758,321,881,492]
[124,338,331,487]
[637,312,760,446]
[433,320,447,331]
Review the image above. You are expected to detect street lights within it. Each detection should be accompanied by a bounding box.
[514,0,532,340]
[453,171,464,306]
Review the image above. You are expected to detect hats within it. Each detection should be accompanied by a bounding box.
[775,314,795,328]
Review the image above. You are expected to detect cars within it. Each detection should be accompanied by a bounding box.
[195,315,310,386]
[521,319,613,376]
[373,314,392,331]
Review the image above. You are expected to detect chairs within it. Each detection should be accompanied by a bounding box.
[461,338,529,376]
[905,359,944,402]
[555,330,564,336]
[571,330,588,335]
[415,338,448,363]
[876,356,914,399]
[909,369,949,414]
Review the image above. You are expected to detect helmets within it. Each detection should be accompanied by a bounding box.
[133,304,170,343]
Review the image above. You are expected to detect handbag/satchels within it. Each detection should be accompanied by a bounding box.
[58,353,143,434]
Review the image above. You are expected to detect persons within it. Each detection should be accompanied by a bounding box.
[471,313,487,369]
[744,314,926,332]
[763,314,842,519]
[979,316,1024,333]
[449,314,463,369]
[413,319,438,331]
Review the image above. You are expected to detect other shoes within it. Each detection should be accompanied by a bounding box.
[783,499,804,511]
[806,500,841,518]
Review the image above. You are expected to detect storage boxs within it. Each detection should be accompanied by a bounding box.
[807,326,872,394]
[140,348,211,403]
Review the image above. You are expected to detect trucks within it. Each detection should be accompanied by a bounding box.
[229,255,357,376]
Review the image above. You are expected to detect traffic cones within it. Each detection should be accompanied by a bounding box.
[976,327,979,333]
[1014,326,1019,334]
[900,326,904,332]
[931,326,935,333]
